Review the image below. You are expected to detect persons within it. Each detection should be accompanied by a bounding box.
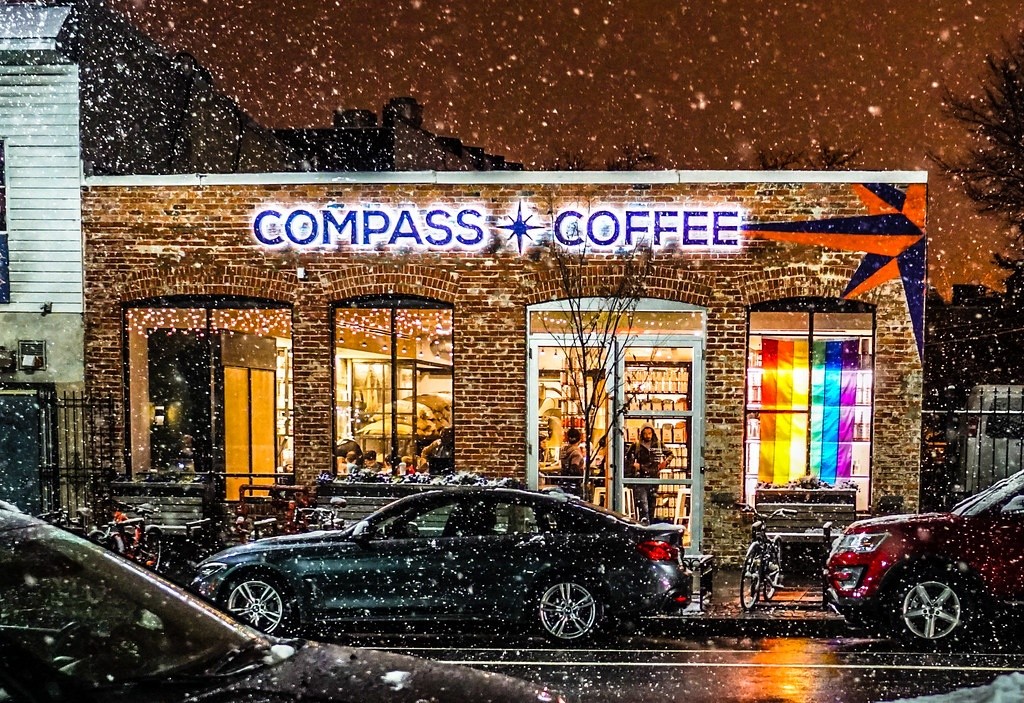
[403,501,498,537]
[279,459,293,483]
[561,429,606,496]
[539,436,550,485]
[337,401,452,476]
[176,434,195,480]
[626,423,674,525]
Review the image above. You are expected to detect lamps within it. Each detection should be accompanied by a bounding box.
[39,301,55,312]
[153,401,165,426]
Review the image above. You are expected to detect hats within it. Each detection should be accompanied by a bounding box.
[568,429,580,444]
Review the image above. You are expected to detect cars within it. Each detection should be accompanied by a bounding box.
[189,486,693,646]
[819,467,1024,644]
[0,499,568,702]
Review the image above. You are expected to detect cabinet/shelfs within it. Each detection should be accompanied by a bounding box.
[624,355,693,524]
[278,346,347,474]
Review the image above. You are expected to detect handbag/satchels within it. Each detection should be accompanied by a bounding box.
[625,442,641,489]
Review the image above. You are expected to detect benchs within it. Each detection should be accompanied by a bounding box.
[753,503,854,582]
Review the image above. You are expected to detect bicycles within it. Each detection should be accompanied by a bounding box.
[27,498,352,583]
[737,502,798,610]
